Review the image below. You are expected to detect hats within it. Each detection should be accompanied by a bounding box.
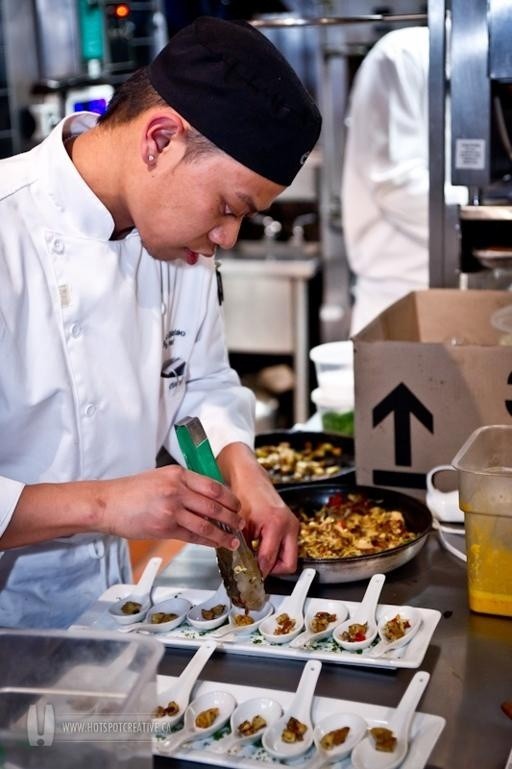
[148,15,322,187]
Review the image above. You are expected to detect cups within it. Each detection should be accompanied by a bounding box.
[452,420,511,618]
[307,341,354,436]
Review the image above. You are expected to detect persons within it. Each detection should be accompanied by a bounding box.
[338,28,430,338]
[1,11,322,628]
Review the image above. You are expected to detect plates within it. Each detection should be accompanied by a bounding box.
[8,667,446,768]
[59,583,441,673]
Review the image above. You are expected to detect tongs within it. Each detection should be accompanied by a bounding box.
[173,413,267,613]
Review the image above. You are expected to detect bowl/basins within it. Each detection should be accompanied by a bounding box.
[240,430,356,483]
[236,484,432,582]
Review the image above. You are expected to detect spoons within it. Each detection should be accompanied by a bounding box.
[103,559,421,665]
[130,641,436,769]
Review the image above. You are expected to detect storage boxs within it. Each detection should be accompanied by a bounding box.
[351,289,511,503]
